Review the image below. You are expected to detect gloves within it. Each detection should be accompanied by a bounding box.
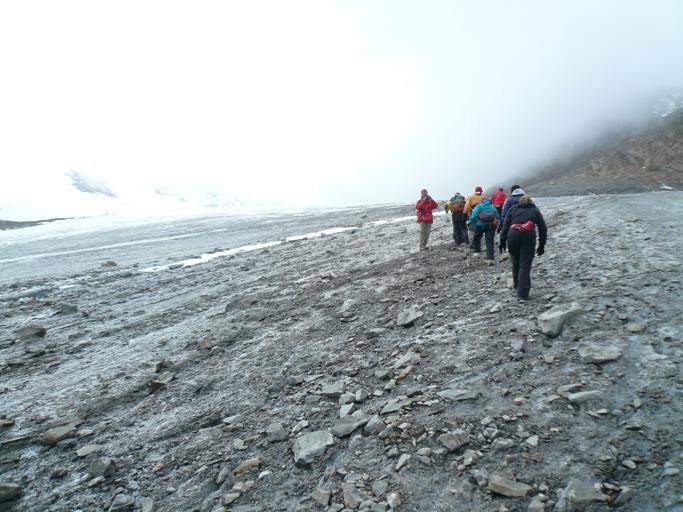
[536,244,546,256]
[498,240,506,252]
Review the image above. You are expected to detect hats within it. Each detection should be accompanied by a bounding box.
[474,187,483,193]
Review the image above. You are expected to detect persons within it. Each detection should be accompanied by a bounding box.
[498,195,547,298]
[415,188,437,252]
[465,198,499,265]
[461,186,485,219]
[490,188,506,216]
[444,193,469,246]
[496,184,526,230]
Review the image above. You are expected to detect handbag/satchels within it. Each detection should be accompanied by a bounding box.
[421,214,434,224]
[478,211,495,221]
[511,221,534,232]
